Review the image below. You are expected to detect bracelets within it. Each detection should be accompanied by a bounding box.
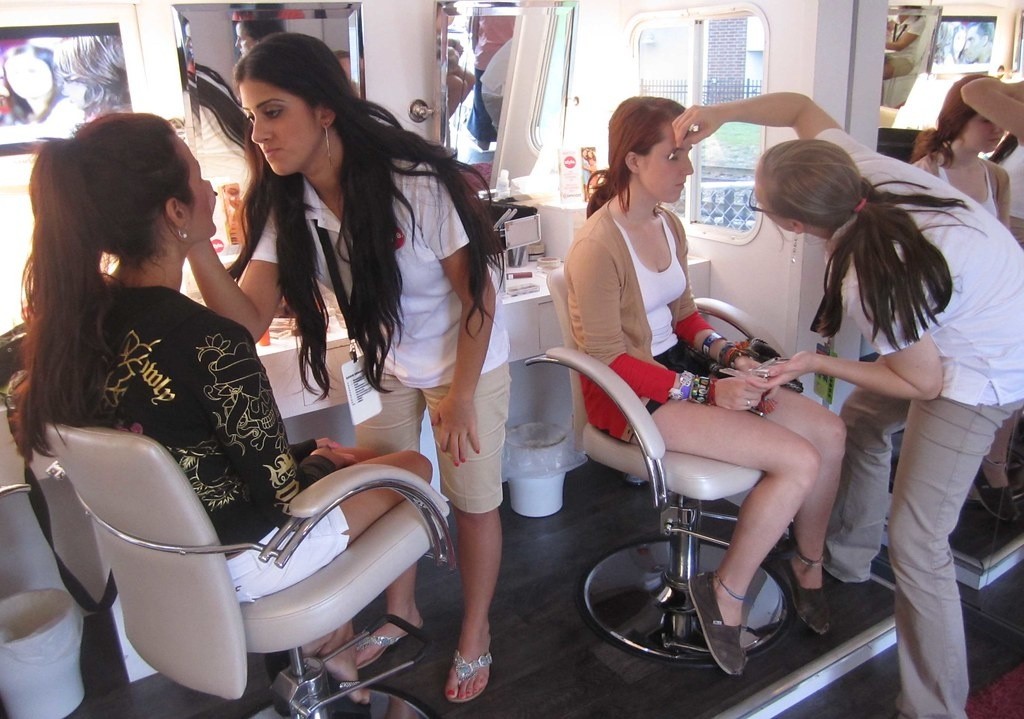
[668,370,711,404]
[701,332,747,368]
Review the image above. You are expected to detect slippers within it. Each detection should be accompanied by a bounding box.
[354,617,424,669]
[444,647,492,703]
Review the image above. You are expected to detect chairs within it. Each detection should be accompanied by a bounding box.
[525,266,800,667]
[42,418,457,719]
[876,128,921,165]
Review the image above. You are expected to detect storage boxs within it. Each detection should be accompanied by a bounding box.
[504,214,542,251]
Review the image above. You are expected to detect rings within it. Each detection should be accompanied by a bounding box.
[746,400,751,407]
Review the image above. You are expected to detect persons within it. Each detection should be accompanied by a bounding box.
[910,74,1024,518]
[435,0,517,153]
[0,33,132,139]
[186,32,518,702]
[565,94,845,679]
[883,5,926,80]
[936,20,991,66]
[670,91,1024,719]
[10,110,435,713]
[185,19,353,231]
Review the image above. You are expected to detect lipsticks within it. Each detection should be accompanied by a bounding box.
[505,272,532,279]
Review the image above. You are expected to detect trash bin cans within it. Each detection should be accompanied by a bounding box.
[505,422,569,518]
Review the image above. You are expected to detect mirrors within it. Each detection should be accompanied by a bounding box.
[170,2,366,188]
[821,1,1024,627]
[436,0,580,202]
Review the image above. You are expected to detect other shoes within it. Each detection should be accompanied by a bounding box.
[972,464,1020,521]
[777,559,832,634]
[686,571,747,675]
[819,564,878,603]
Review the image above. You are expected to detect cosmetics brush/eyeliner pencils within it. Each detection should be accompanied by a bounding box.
[669,124,694,159]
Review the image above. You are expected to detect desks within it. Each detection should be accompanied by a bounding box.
[0,259,709,482]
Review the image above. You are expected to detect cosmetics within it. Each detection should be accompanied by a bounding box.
[751,356,792,378]
[486,169,518,230]
[501,280,540,302]
[258,304,349,347]
[536,256,562,270]
